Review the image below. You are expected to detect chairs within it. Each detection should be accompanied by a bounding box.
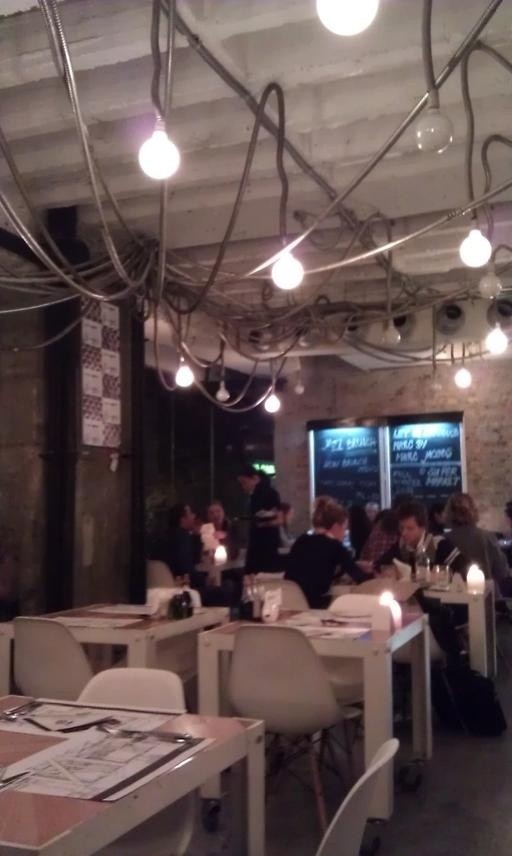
[3,528,511,853]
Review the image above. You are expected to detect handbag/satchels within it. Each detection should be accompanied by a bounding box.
[431,667,507,737]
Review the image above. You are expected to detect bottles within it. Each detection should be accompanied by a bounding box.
[170,574,187,619]
[237,574,260,621]
[183,576,194,617]
[415,544,430,583]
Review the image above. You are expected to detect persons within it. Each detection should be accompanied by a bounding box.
[284,495,370,611]
[277,502,298,550]
[234,461,283,574]
[346,490,512,598]
[154,499,241,603]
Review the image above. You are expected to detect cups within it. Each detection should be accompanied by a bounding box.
[435,564,449,588]
[354,560,373,574]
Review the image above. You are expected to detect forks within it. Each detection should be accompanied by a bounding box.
[0,702,42,721]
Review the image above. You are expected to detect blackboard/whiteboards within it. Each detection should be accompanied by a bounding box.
[384,410,468,510]
[305,414,384,534]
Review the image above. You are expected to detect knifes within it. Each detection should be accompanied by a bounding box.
[97,722,192,744]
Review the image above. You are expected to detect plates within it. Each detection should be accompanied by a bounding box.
[337,609,371,617]
